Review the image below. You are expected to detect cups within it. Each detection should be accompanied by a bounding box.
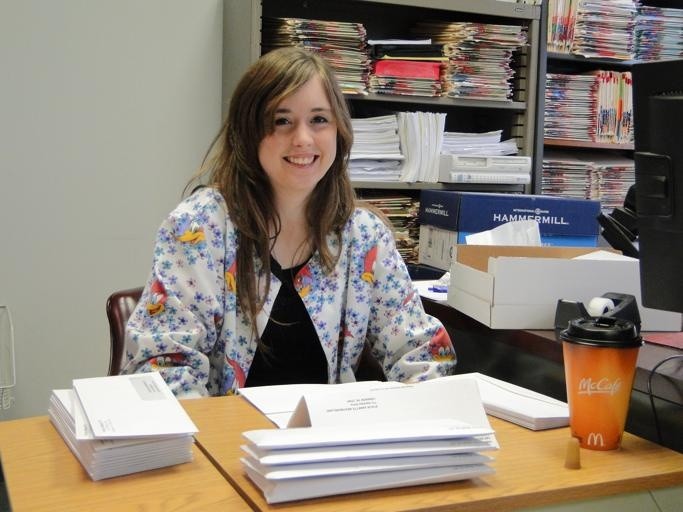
[559,316,646,453]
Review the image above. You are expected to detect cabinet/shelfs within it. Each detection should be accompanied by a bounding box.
[423,295,682,457]
[219,0,541,283]
[533,1,681,206]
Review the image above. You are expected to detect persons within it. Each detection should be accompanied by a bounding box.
[118,48,457,400]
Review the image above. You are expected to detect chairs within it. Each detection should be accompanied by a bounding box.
[104,284,146,377]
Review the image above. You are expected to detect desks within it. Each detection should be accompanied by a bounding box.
[0,381,680,511]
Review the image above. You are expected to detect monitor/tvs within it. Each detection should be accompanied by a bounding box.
[632,59,683,319]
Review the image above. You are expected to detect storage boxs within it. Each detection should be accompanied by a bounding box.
[414,186,602,278]
[445,240,644,331]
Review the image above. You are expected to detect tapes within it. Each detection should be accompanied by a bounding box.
[586,296,616,318]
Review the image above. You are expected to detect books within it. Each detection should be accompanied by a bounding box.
[262,1,681,259]
